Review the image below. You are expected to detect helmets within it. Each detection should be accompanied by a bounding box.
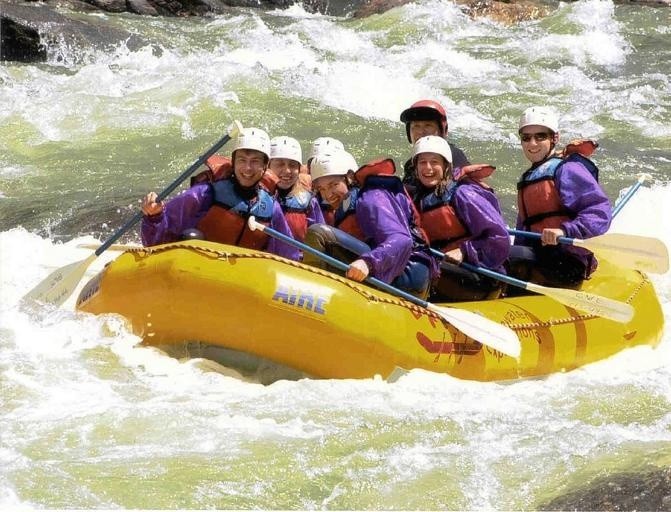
[400,100,452,164]
[519,106,557,133]
[232,128,359,181]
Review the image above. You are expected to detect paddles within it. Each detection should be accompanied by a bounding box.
[248,216,521,358]
[429,247,634,323]
[18,118,243,322]
[509,229,669,274]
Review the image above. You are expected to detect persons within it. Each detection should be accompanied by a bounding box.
[268,135,326,262]
[411,135,511,304]
[300,137,345,192]
[509,106,612,285]
[399,100,471,200]
[141,127,301,262]
[310,149,442,303]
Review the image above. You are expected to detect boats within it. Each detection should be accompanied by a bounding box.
[77,238,663,382]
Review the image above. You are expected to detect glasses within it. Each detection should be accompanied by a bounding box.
[519,133,547,142]
